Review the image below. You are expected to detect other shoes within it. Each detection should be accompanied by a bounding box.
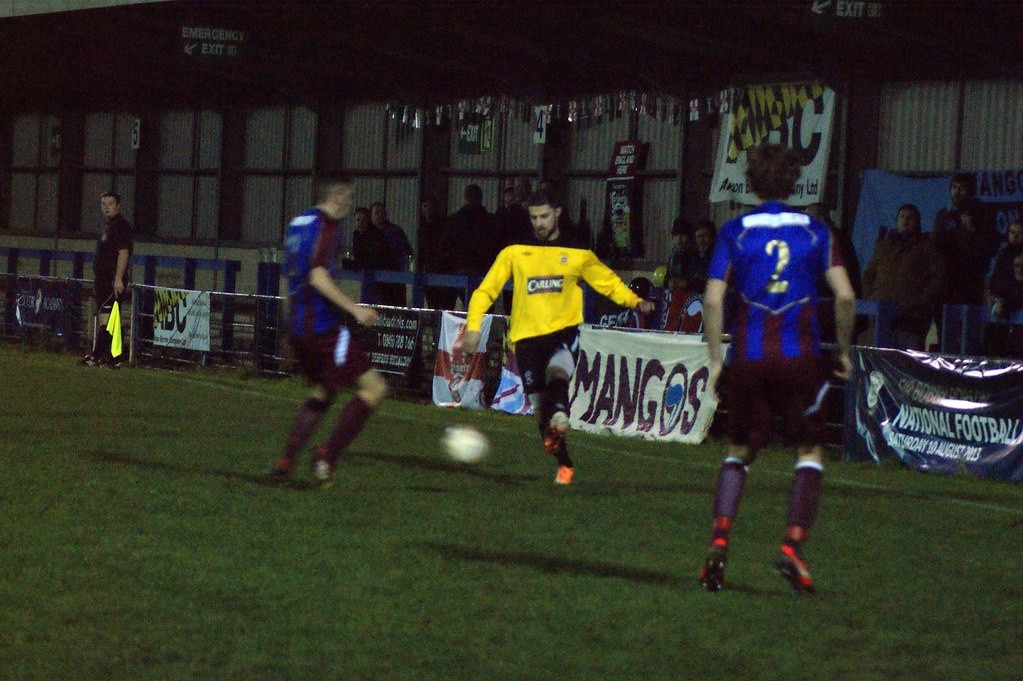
[271,466,290,484]
[310,446,333,488]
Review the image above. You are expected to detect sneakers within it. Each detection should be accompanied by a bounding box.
[543,412,569,454]
[699,538,728,591]
[101,358,120,369]
[553,466,574,485]
[77,355,108,367]
[778,544,816,594]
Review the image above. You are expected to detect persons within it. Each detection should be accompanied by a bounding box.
[464,187,654,485]
[77,190,133,369]
[699,144,853,594]
[349,171,1023,363]
[269,171,388,494]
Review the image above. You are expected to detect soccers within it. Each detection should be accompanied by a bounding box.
[445,426,488,465]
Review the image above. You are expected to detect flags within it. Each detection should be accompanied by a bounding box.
[106,301,122,358]
